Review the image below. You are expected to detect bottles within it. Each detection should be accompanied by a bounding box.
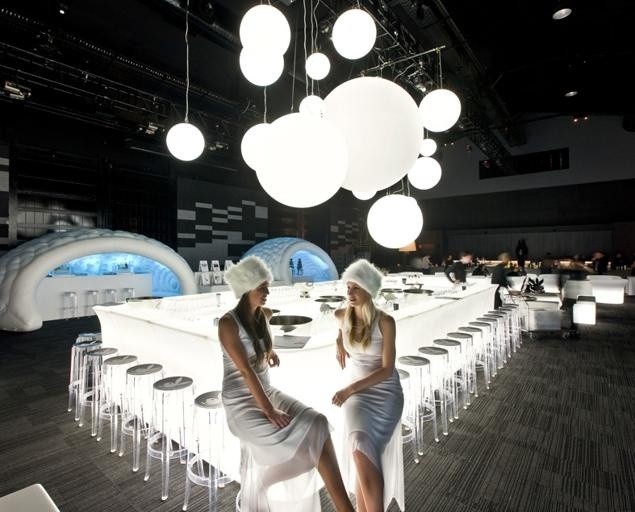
[62,287,136,309]
[297,258,303,276]
[289,257,295,276]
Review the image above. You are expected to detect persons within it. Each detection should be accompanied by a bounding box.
[217,254,355,511]
[593,249,608,275]
[296,258,303,275]
[491,252,510,310]
[444,254,453,272]
[333,257,404,511]
[444,250,474,289]
[289,258,294,269]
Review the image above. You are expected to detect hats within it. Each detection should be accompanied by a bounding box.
[342,259,381,298]
[224,256,272,298]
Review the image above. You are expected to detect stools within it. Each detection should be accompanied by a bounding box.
[179,390,243,511]
[68,329,164,475]
[60,288,135,324]
[391,302,523,465]
[142,375,196,503]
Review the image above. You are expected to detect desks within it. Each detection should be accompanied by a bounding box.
[1,482,59,512]
[92,275,500,512]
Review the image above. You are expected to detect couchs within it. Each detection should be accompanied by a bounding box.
[409,250,635,340]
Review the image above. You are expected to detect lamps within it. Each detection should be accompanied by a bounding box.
[165,1,462,250]
